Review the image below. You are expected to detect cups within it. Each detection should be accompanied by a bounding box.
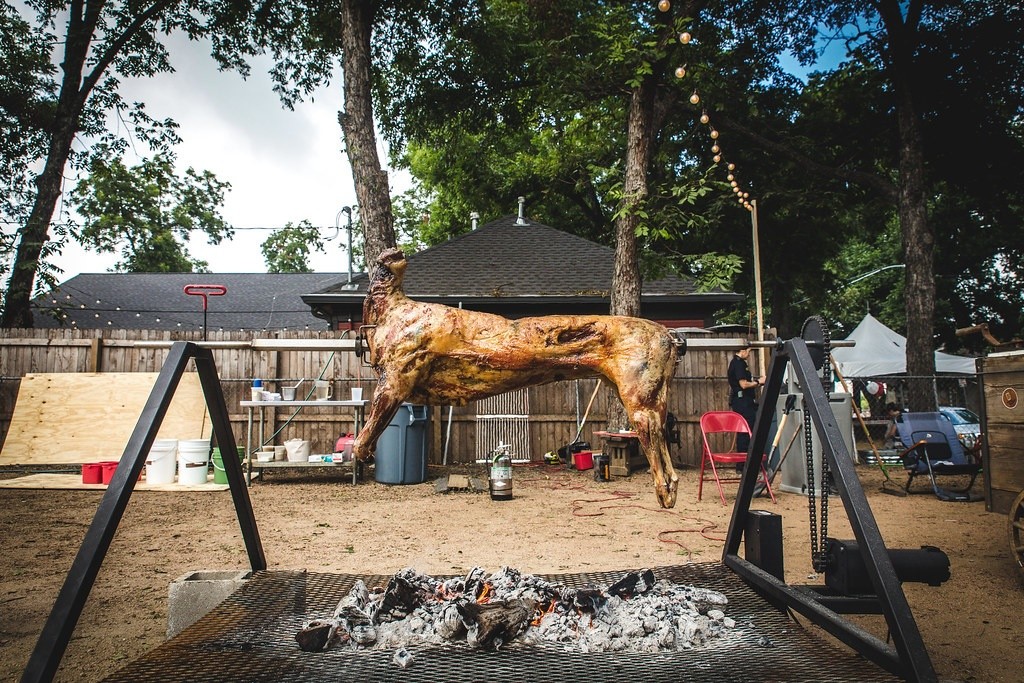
[262,446,274,452]
[251,387,264,401]
[254,379,264,387]
[352,388,361,401]
[274,446,286,461]
[281,387,297,401]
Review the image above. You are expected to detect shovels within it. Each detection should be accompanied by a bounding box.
[558,379,601,463]
[752,395,797,497]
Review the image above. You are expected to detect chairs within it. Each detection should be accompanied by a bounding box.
[697,411,777,508]
[896,411,984,500]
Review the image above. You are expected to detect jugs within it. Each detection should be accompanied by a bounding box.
[316,380,333,401]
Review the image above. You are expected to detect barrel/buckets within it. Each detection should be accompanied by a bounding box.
[82,462,142,485]
[594,454,610,481]
[177,439,211,485]
[286,440,309,462]
[145,439,177,485]
[573,451,593,471]
[211,446,245,484]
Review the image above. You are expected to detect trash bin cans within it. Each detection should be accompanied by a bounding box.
[373,403,433,485]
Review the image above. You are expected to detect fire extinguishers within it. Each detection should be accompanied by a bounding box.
[485,440,514,500]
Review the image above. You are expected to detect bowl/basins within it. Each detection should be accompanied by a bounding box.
[254,452,274,462]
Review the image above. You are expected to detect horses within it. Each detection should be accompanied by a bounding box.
[352,247,679,509]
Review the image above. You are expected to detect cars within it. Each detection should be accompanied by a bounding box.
[937,405,983,464]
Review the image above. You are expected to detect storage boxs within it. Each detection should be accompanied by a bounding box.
[570,449,602,465]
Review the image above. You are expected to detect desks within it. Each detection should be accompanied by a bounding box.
[239,400,367,485]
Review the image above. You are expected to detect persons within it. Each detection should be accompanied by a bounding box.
[885,401,908,438]
[727,346,766,474]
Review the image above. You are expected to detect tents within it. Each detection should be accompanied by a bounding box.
[783,313,980,376]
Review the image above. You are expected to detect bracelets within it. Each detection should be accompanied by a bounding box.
[754,379,759,386]
